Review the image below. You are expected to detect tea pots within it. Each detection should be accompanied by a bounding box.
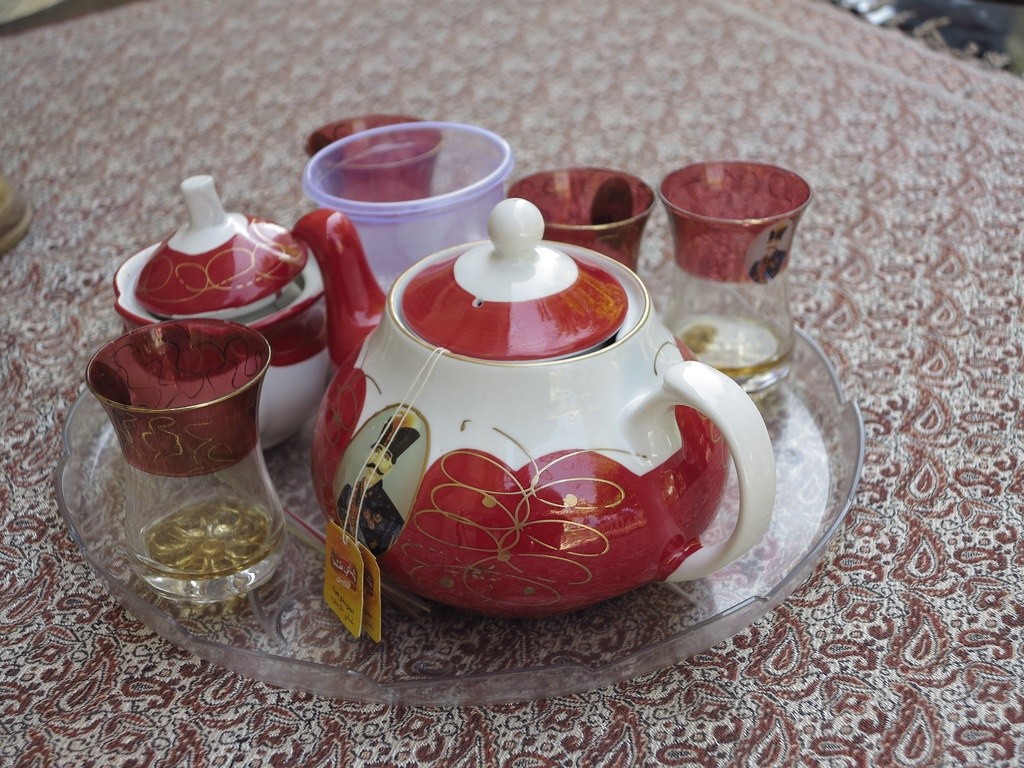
[294,197,778,616]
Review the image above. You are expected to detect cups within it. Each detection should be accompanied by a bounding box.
[655,158,815,404]
[83,318,286,604]
[112,175,332,452]
[298,121,516,290]
[305,114,445,201]
[505,166,660,275]
